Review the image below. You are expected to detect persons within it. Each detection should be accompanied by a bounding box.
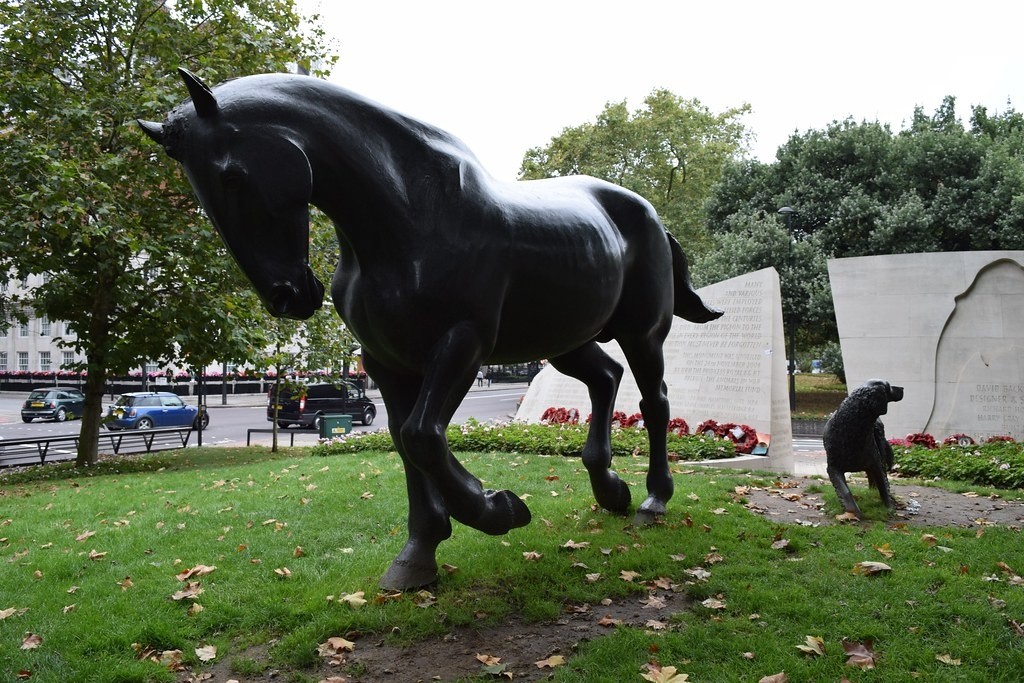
[485,369,493,387]
[477,370,484,387]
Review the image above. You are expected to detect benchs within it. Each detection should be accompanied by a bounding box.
[1,424,194,466]
[246,428,320,447]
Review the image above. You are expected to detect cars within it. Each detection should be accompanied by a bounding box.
[105,391,210,431]
[20,386,87,423]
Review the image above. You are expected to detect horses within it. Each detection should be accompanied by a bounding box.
[139,66,725,589]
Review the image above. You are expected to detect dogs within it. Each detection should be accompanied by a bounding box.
[822,379,905,519]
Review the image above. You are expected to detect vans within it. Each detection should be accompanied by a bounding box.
[266,379,377,430]
